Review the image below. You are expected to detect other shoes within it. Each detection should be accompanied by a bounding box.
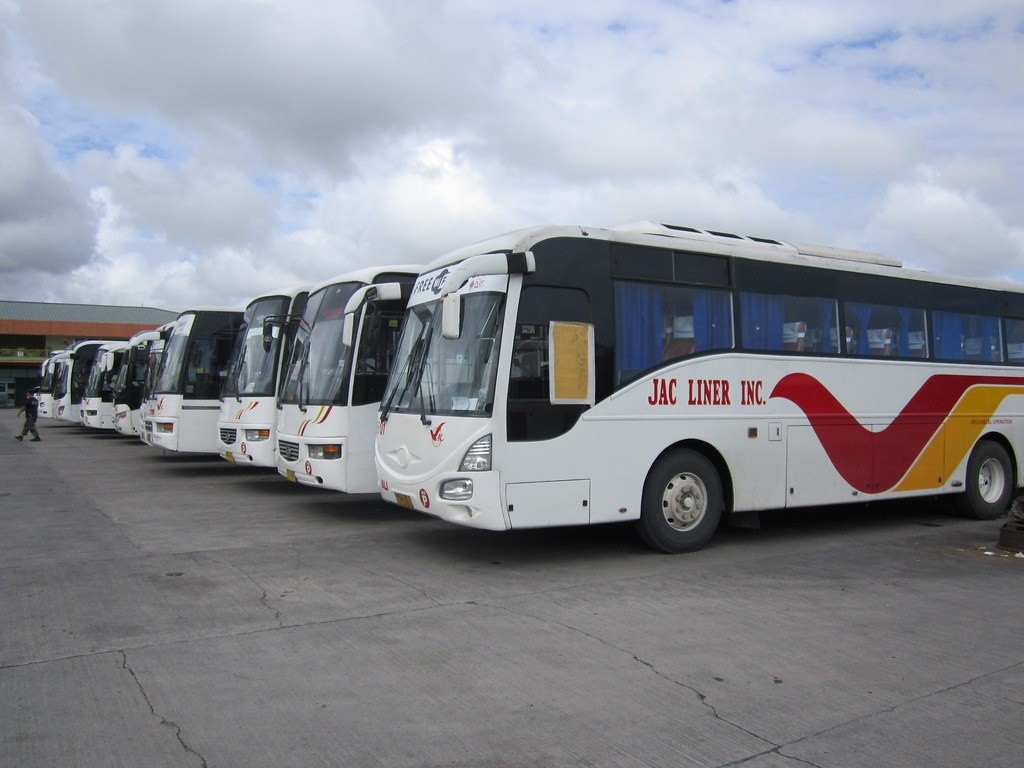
[30,436,40,441]
[15,435,23,441]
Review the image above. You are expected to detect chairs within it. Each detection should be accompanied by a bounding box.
[655,313,1024,372]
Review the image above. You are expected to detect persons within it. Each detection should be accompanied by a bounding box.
[14,392,40,441]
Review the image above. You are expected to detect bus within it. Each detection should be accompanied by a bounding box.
[31,319,178,439]
[261,263,429,496]
[216,280,314,468]
[340,219,1024,553]
[122,306,246,460]
